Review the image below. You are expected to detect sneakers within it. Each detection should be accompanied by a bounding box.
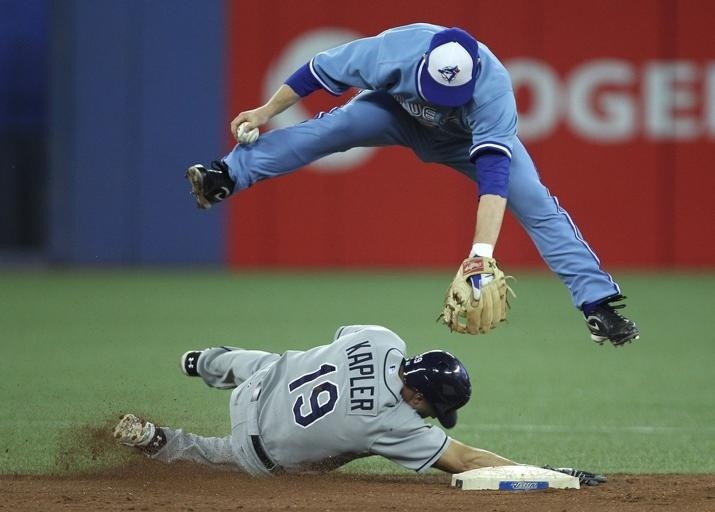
[583,303,640,348]
[112,414,168,455]
[180,350,203,376]
[185,158,235,210]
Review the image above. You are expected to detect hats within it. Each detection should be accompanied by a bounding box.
[420,25,482,108]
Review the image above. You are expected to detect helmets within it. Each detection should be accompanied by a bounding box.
[403,349,471,429]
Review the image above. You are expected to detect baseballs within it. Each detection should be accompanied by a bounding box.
[236,120,259,146]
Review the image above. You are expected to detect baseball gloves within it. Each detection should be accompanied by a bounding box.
[442,254,517,335]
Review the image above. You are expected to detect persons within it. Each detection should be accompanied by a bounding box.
[185,22,640,348]
[112,325,607,486]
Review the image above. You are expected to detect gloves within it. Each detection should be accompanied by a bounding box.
[541,464,609,488]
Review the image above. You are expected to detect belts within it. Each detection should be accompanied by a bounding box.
[249,381,284,476]
